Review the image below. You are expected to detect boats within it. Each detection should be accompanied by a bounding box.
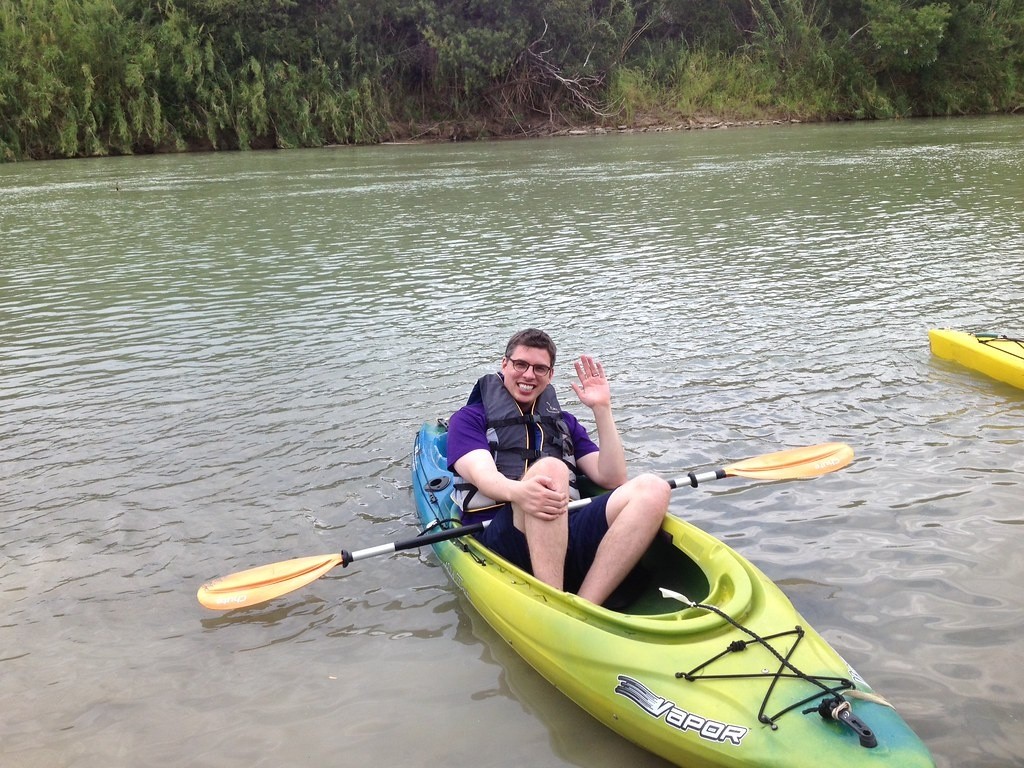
[413,421,936,768]
[929,329,1024,393]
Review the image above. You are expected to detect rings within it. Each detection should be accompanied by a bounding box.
[592,373,599,377]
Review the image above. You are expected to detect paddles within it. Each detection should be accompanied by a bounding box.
[196,442,854,612]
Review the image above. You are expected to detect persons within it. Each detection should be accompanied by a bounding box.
[447,328,672,607]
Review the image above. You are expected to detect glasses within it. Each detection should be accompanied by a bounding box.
[506,355,553,376]
